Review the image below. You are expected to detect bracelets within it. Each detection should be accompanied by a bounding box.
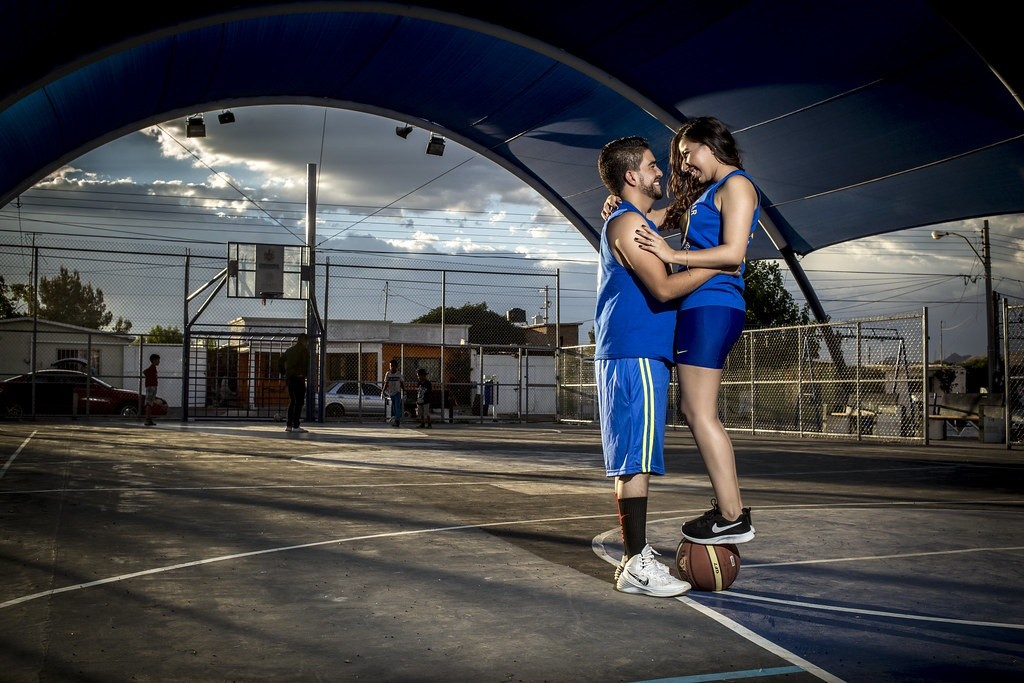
[684,242,692,276]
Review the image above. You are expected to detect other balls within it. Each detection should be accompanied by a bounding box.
[389,416,396,425]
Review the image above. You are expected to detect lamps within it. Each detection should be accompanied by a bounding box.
[184,115,207,138]
[218,111,235,125]
[425,137,445,156]
[396,126,413,139]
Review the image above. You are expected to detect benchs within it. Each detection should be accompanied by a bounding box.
[929,393,1006,443]
[827,392,905,435]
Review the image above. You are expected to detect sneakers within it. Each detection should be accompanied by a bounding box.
[617,543,691,597]
[684,498,755,533]
[681,510,756,544]
[615,546,679,583]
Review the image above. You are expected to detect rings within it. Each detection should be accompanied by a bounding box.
[649,239,653,245]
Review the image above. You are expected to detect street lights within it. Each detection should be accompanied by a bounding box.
[930,229,997,406]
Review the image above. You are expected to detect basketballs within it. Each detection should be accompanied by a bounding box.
[674,537,741,593]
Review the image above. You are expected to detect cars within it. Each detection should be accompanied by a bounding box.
[0,368,168,421]
[313,380,390,417]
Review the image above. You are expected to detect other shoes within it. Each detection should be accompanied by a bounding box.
[292,427,308,432]
[284,427,292,432]
[144,419,156,426]
[416,425,425,428]
[392,420,400,427]
[426,426,432,429]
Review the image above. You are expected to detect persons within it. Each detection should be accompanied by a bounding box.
[594,118,757,598]
[142,354,160,426]
[278,332,309,431]
[379,360,433,429]
